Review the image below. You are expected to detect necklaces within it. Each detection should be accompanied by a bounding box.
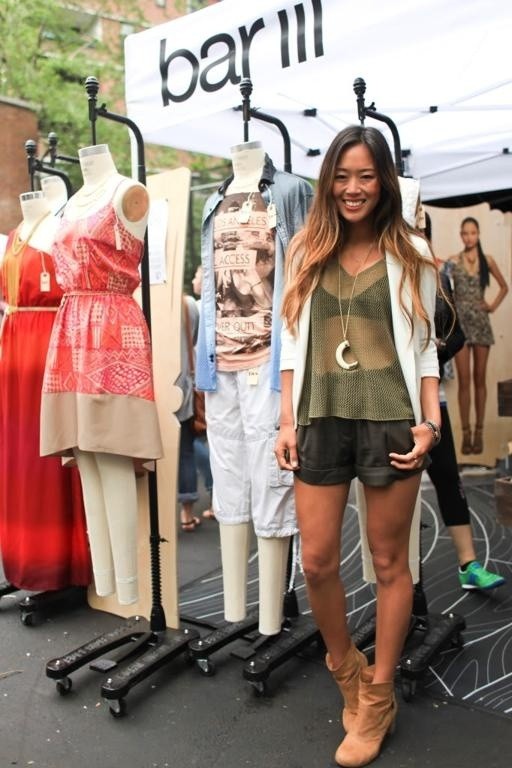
[333,232,378,370]
[6,210,52,315]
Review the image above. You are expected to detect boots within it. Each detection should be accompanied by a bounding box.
[326,637,369,731]
[336,664,396,767]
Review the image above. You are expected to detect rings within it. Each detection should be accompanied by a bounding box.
[413,453,417,459]
[414,460,418,463]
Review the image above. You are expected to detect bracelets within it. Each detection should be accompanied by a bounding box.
[424,420,442,444]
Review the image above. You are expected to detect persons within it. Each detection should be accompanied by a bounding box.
[169,291,200,532]
[40,176,68,217]
[0,191,91,600]
[273,126,444,768]
[191,266,217,522]
[417,212,506,589]
[195,140,318,636]
[38,142,164,608]
[446,218,510,456]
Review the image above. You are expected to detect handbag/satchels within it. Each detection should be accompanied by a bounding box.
[434,307,467,364]
[192,376,206,436]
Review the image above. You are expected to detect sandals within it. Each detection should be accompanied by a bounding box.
[461,424,484,454]
[181,517,199,531]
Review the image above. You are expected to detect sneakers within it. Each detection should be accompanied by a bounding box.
[458,561,505,591]
